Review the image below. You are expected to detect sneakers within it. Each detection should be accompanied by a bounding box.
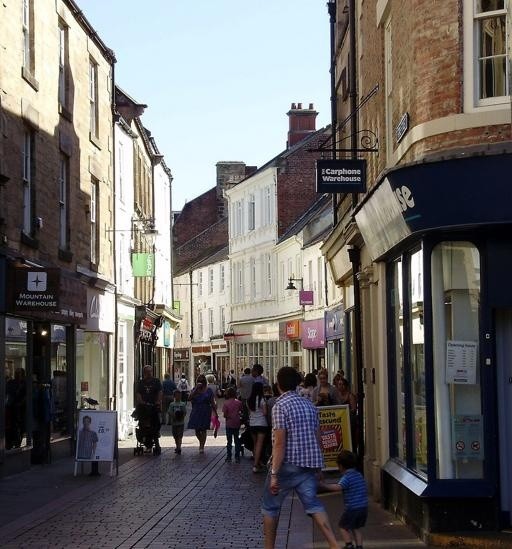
[343,544,354,549]
[199,448,204,454]
[252,465,264,473]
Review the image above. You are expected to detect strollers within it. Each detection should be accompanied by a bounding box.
[132,402,162,457]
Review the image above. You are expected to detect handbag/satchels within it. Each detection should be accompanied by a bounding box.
[249,396,269,427]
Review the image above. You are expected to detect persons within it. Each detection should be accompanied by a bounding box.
[78,416,99,460]
[205,375,222,430]
[246,382,269,473]
[221,388,244,464]
[188,375,219,454]
[260,367,340,549]
[253,364,269,388]
[313,368,337,406]
[21,377,54,466]
[333,370,357,413]
[298,369,318,398]
[177,375,190,402]
[267,383,280,410]
[227,369,236,387]
[131,365,163,453]
[4,367,28,448]
[160,374,178,425]
[167,390,187,455]
[240,368,256,400]
[319,448,370,549]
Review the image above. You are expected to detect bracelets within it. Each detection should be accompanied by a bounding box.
[269,472,280,477]
